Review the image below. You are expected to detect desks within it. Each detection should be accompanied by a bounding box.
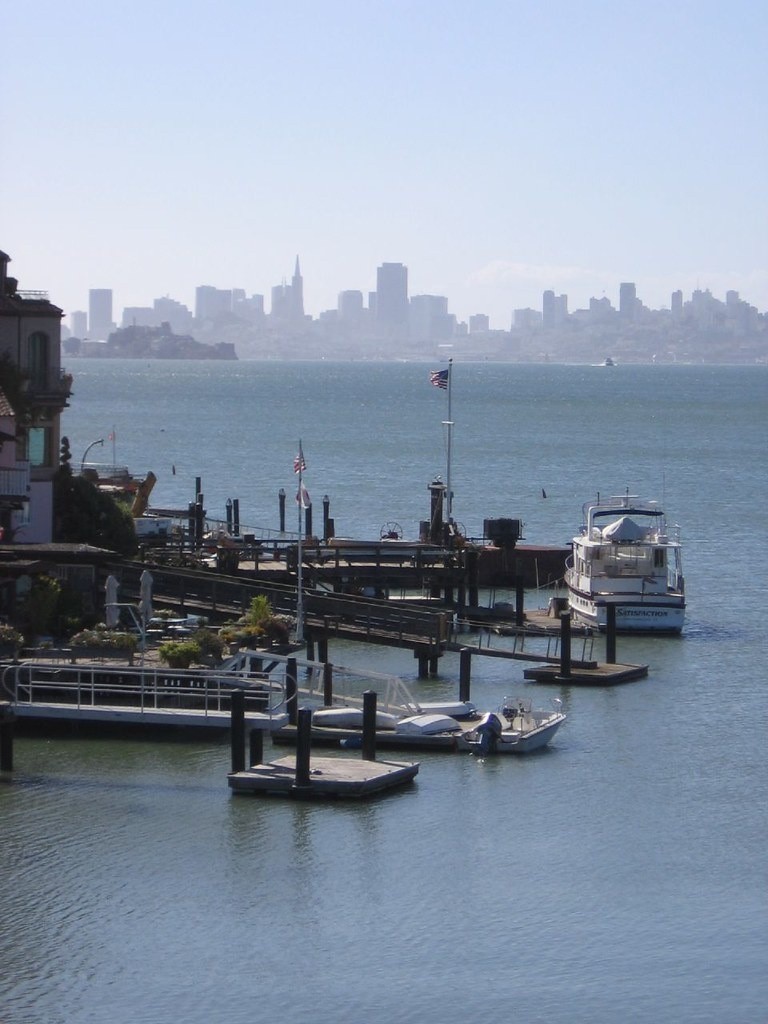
[150,618,187,626]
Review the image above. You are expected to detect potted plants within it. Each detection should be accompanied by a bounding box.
[159,595,298,669]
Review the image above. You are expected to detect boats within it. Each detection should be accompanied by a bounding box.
[604,357,615,367]
[560,486,689,636]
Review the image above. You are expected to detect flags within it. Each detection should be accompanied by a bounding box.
[430,369,447,389]
[106,431,114,442]
[293,446,307,474]
[295,479,311,509]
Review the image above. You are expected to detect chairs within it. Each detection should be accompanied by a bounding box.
[502,696,532,734]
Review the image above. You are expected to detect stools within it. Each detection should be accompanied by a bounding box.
[146,626,222,643]
